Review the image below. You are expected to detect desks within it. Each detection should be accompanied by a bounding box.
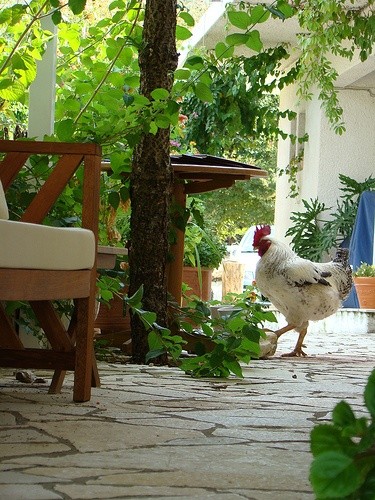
[101,163,268,358]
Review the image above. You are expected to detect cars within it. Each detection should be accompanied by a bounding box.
[212,245,237,280]
[238,224,274,292]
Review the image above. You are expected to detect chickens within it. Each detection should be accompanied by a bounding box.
[252,223,353,357]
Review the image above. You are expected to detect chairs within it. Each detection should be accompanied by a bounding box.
[0,140,102,404]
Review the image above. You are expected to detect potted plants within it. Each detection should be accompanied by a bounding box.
[352,261,375,309]
